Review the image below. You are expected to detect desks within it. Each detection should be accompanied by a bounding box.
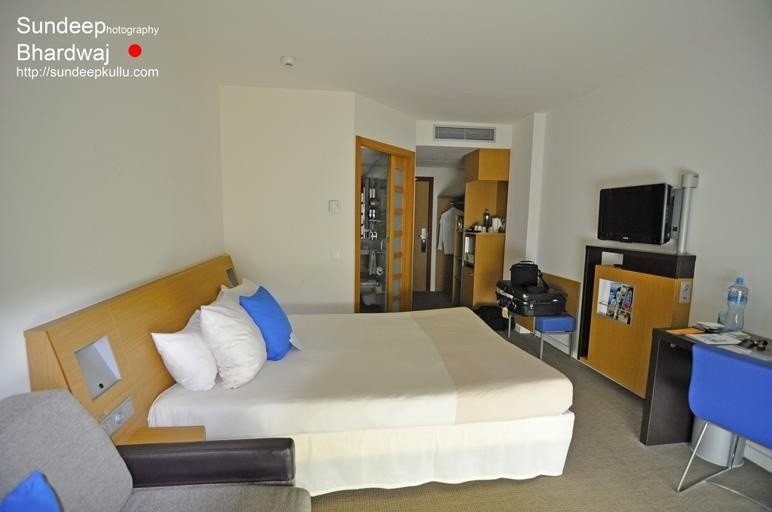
[639,324,771,448]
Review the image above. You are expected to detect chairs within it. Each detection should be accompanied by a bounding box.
[674,343,771,512]
[2,389,312,510]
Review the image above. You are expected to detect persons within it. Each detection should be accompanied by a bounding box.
[605,285,634,324]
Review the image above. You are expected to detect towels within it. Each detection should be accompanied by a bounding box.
[368,249,376,275]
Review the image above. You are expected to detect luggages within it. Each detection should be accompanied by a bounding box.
[495,279,569,317]
[472,305,515,332]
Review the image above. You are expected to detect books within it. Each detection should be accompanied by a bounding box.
[684,321,751,345]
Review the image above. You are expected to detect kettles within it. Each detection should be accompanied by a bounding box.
[491,217,502,232]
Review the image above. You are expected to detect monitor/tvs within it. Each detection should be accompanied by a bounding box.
[597,182,674,246]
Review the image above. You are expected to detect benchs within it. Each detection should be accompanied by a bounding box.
[507,272,581,361]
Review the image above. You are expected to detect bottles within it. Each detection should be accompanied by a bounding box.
[483,209,490,232]
[725,276,749,331]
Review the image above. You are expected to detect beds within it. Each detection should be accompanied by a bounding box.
[21,253,576,495]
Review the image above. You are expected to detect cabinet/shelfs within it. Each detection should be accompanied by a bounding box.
[435,149,512,310]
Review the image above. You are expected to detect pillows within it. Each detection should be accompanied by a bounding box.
[238,285,292,361]
[2,470,64,511]
[220,279,258,302]
[150,306,217,391]
[198,289,269,388]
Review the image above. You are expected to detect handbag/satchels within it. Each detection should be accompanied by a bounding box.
[509,260,539,288]
